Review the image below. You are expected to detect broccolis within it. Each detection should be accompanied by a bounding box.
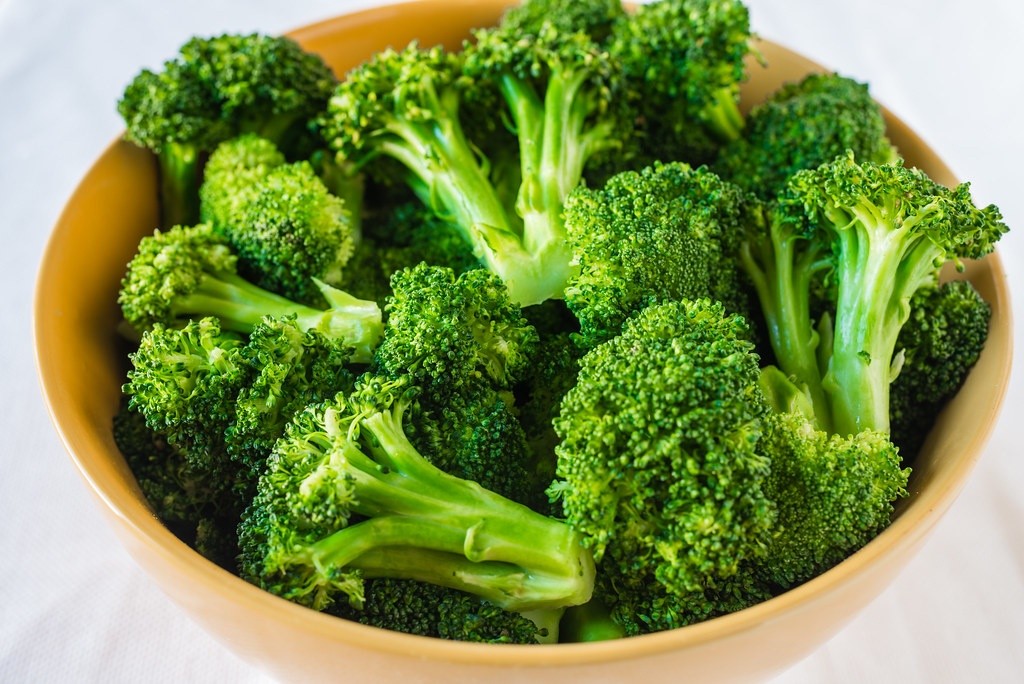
[117,3,1007,637]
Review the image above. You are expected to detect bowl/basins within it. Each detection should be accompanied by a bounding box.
[36,0,1013,684]
